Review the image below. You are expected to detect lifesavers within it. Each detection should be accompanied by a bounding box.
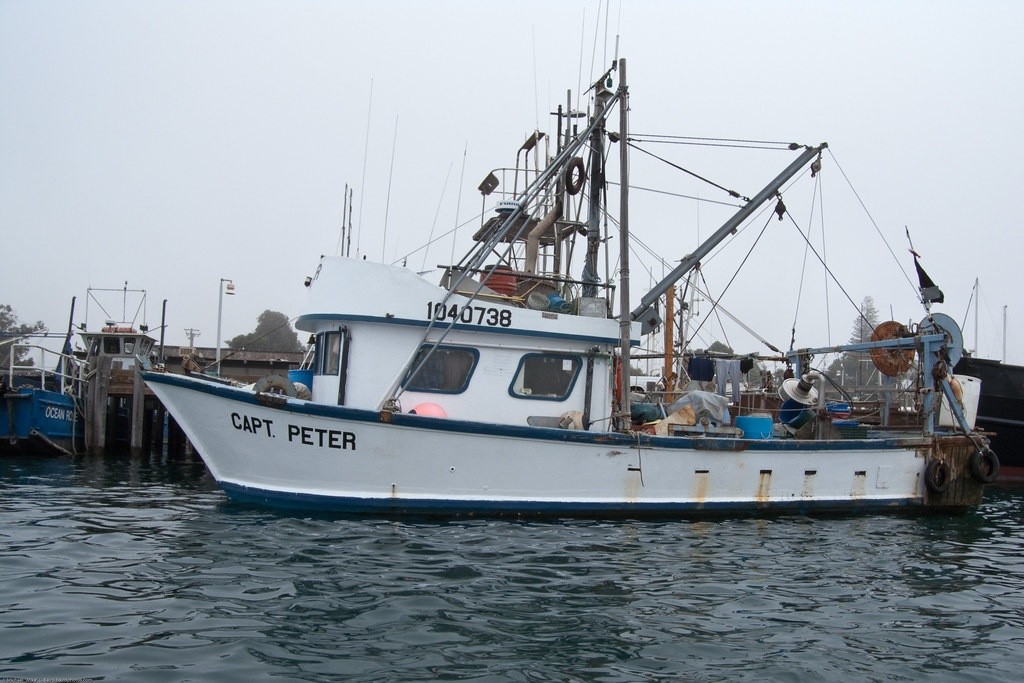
[971,447,1001,485]
[924,460,951,493]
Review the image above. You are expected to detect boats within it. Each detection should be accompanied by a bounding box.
[136,57,1001,520]
[952,356,1024,478]
[0,285,158,458]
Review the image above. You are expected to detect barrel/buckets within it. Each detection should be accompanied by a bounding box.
[480,265,517,297]
[288,370,313,393]
[827,403,849,412]
[526,292,550,311]
[938,374,981,430]
[778,399,816,430]
[734,416,773,439]
